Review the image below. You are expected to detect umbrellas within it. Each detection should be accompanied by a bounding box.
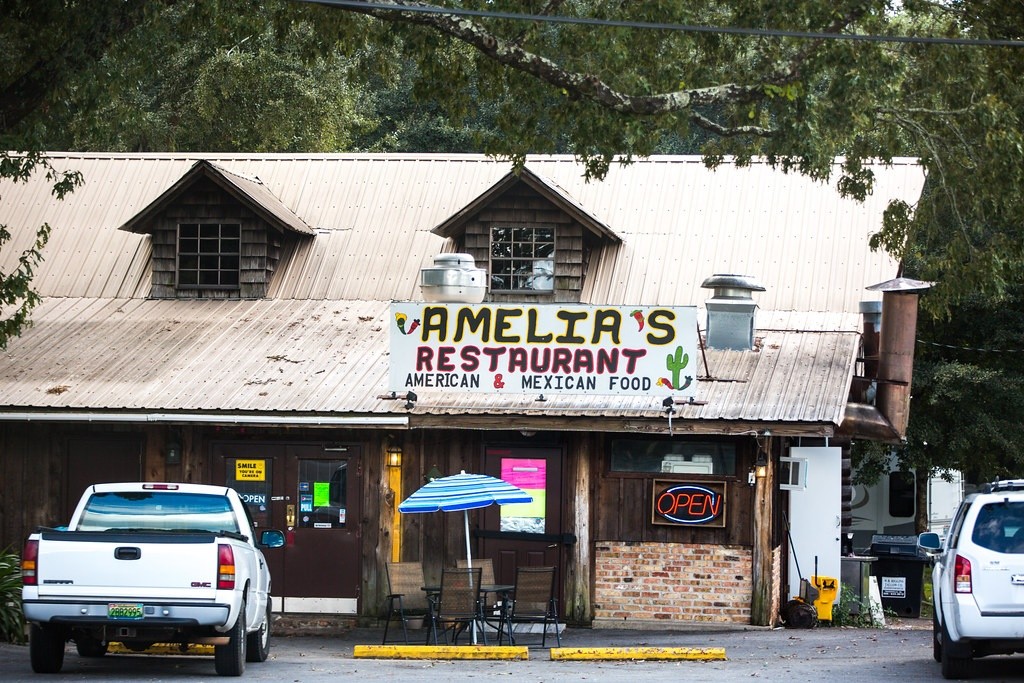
[397,473,534,646]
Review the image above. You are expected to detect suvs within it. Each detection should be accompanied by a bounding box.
[913,476,1024,682]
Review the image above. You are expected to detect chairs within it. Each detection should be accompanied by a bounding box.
[426,568,483,647]
[497,566,563,648]
[383,562,433,645]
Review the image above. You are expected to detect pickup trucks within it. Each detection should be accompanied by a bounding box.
[18,479,289,678]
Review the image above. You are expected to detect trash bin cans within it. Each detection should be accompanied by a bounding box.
[867,534,929,619]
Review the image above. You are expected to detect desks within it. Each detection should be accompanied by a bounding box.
[420,584,513,647]
